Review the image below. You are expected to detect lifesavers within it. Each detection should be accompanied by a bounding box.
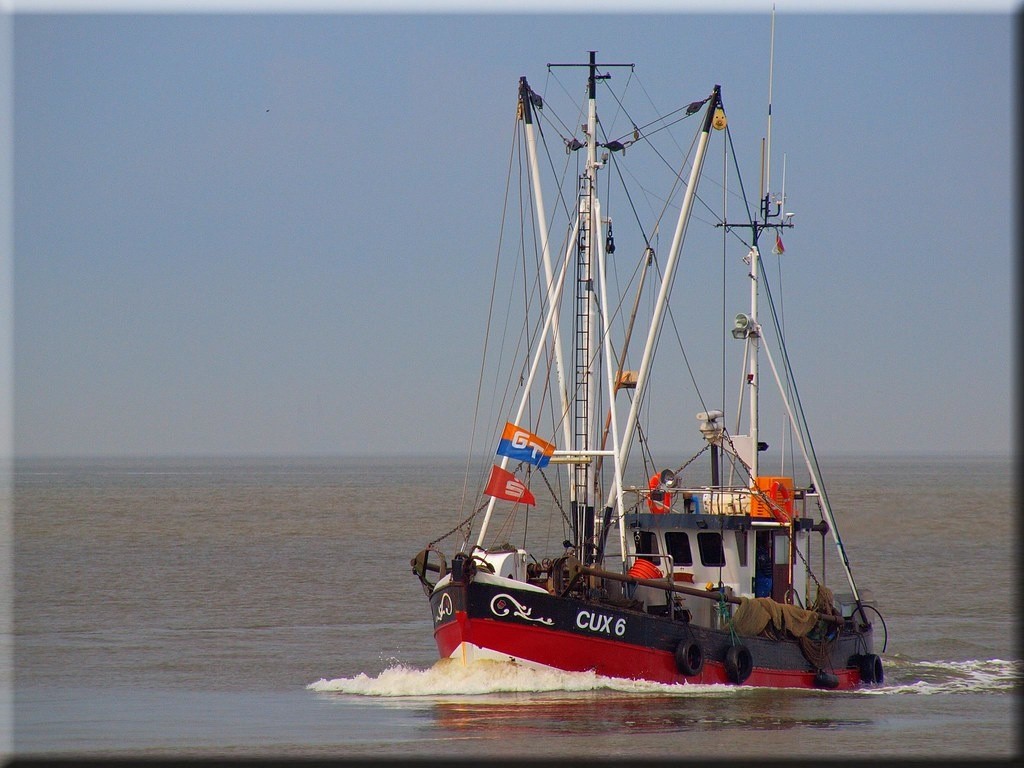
[769,479,794,521]
[673,637,705,676]
[860,653,884,684]
[726,644,755,683]
[646,473,670,514]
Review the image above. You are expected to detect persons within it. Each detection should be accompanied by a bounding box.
[755,542,773,597]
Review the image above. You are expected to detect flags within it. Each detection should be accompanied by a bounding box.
[495,423,557,468]
[483,464,536,508]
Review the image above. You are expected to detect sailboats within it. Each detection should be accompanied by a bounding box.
[409,51,888,693]
